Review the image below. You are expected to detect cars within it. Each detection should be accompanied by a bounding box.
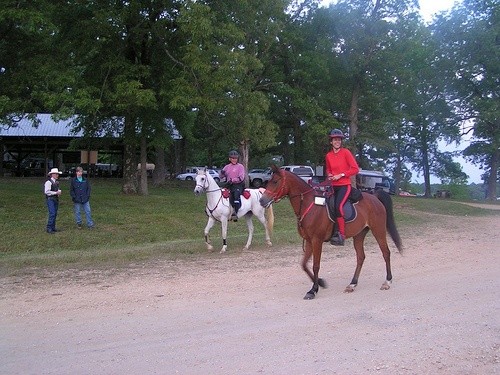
[12,158,49,178]
[64,162,101,177]
[174,164,395,196]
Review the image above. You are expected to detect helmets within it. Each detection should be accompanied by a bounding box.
[229,151,239,158]
[330,129,346,139]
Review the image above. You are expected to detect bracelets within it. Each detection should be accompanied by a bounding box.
[340,173,345,177]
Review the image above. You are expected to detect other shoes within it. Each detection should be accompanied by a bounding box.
[89,226,93,231]
[47,230,54,233]
[78,225,82,230]
[53,229,57,231]
[231,215,237,222]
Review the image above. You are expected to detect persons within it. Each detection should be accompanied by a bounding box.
[70,167,95,229]
[325,129,359,246]
[44,168,62,233]
[220,151,246,222]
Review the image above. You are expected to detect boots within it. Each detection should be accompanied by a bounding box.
[332,217,344,246]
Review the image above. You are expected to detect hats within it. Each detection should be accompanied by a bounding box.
[47,168,62,176]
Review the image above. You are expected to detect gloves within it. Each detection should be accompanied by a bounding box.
[225,181,231,188]
[222,170,225,174]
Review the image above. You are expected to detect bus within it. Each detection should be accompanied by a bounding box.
[95,163,114,176]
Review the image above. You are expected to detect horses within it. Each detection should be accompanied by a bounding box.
[258,159,405,300]
[192,165,277,254]
